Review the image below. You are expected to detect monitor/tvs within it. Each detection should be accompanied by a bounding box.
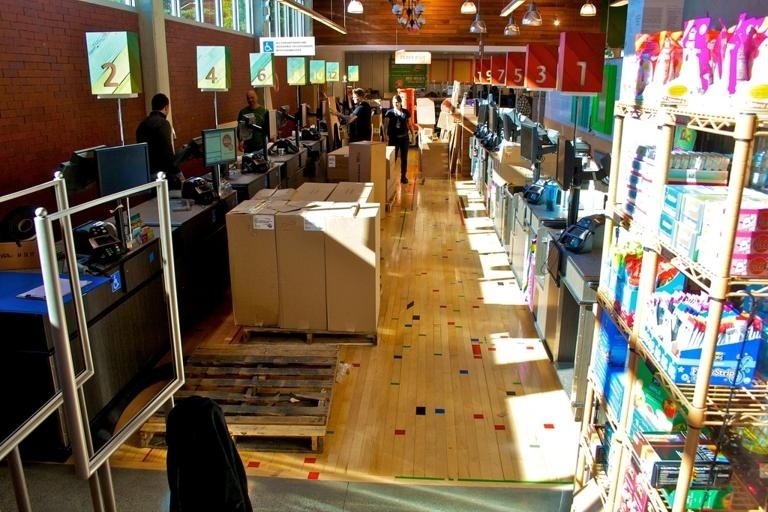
[237,112,256,139]
[489,107,503,133]
[276,105,290,128]
[520,123,542,163]
[504,113,517,141]
[316,100,327,120]
[478,105,488,125]
[555,135,583,191]
[175,134,203,168]
[94,142,153,198]
[474,98,479,117]
[69,144,106,191]
[295,103,307,128]
[201,128,237,168]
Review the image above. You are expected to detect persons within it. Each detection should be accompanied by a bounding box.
[134,92,199,194]
[384,94,415,184]
[236,90,270,153]
[342,83,356,113]
[468,84,531,119]
[333,88,372,144]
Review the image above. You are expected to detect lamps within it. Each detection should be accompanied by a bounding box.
[344,2,630,34]
